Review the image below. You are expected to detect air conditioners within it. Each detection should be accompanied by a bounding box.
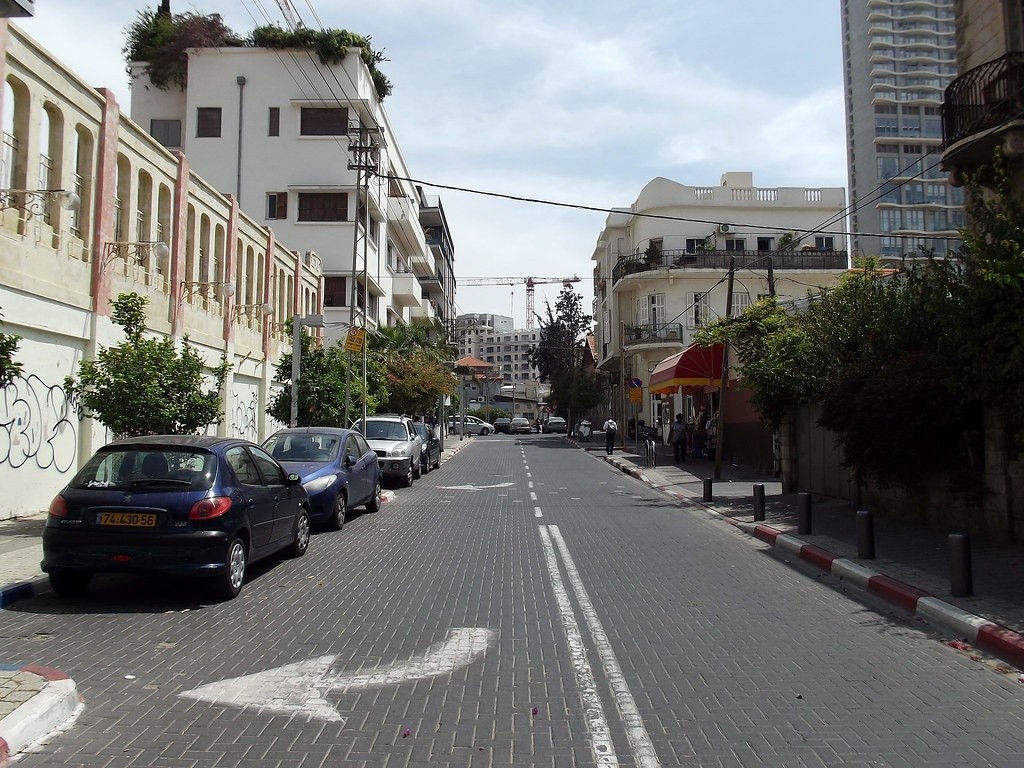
[719,223,735,234]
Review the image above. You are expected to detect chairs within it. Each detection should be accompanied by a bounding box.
[289,438,313,459]
[141,454,167,480]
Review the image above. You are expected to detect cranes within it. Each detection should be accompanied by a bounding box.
[453,274,582,329]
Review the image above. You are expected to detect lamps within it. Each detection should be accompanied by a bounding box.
[0,186,81,226]
[100,241,169,275]
[232,303,273,323]
[178,281,234,305]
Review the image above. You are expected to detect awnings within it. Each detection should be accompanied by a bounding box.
[648,340,730,394]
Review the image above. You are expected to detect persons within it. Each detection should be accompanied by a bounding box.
[603,416,617,455]
[536,418,540,434]
[667,413,690,464]
[425,410,435,430]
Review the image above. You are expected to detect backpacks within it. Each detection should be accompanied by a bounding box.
[607,420,616,435]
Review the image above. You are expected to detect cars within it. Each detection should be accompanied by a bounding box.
[412,421,441,475]
[448,413,495,436]
[349,413,423,487]
[38,434,311,601]
[493,418,512,435]
[542,416,567,433]
[510,416,531,435]
[258,426,383,530]
